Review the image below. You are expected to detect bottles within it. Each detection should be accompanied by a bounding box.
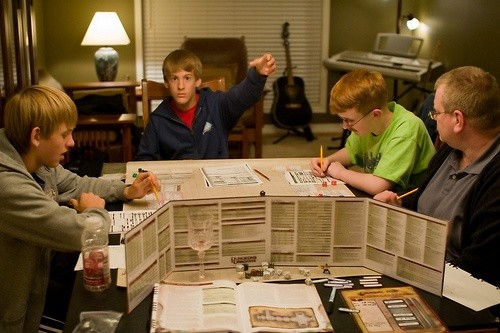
[82,216,109,291]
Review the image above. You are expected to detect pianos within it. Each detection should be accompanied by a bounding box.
[323,48,445,154]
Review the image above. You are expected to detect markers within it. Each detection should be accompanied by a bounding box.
[327,288,337,313]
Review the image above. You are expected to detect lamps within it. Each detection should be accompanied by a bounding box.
[81,10,131,82]
[406,14,420,31]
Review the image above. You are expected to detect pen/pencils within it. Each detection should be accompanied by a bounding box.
[320,144,323,176]
[397,187,418,199]
[150,182,159,199]
[254,169,269,180]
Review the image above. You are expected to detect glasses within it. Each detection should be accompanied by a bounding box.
[427,110,463,120]
[338,107,381,131]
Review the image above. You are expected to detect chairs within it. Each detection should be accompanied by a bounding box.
[141,34,270,161]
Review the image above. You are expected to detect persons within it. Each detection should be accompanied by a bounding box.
[0,85,161,333]
[136,48,276,161]
[372,65,500,333]
[312,69,437,194]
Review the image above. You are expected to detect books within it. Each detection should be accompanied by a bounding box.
[150,280,334,333]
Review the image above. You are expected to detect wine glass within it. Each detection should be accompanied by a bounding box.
[188,211,214,281]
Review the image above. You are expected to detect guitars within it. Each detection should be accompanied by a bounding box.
[269,22,313,131]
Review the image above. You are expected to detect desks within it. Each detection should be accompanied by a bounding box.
[73,113,137,162]
[62,80,141,114]
[60,158,500,333]
